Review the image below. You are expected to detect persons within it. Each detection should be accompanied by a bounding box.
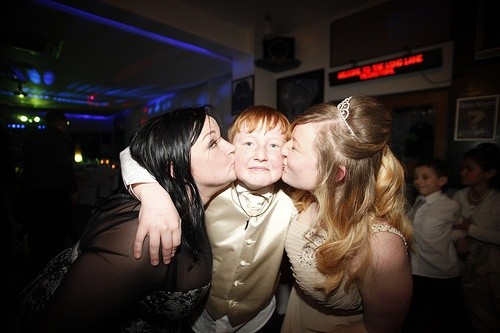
[119,105,299,333]
[450,143,500,333]
[280,93,416,333]
[22,110,79,280]
[0,103,238,333]
[403,159,470,333]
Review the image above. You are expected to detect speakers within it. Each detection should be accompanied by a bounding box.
[262,37,295,66]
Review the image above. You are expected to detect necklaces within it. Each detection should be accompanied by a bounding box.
[470,191,490,203]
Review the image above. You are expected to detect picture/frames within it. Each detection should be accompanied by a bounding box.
[453,95,499,142]
[229,74,255,114]
